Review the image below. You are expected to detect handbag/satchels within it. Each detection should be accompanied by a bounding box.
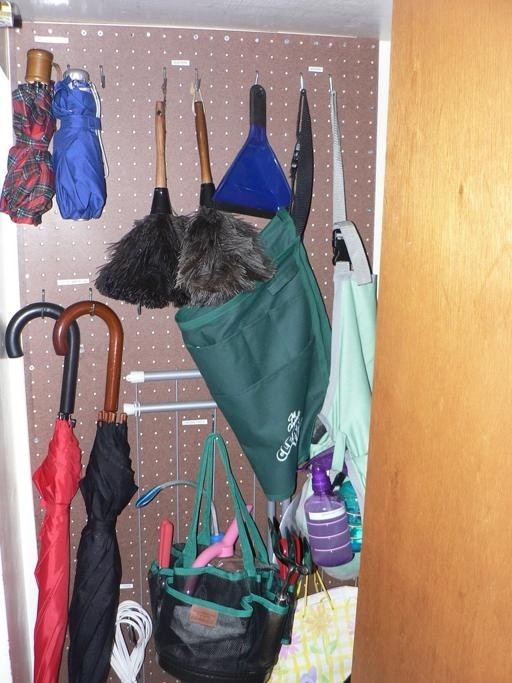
[267,569,358,683]
[146,542,298,683]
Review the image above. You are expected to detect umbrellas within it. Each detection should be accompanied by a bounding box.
[5,301,83,683]
[52,299,140,683]
[0,50,57,227]
[51,69,109,221]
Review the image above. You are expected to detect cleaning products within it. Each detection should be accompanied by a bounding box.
[297,445,354,570]
